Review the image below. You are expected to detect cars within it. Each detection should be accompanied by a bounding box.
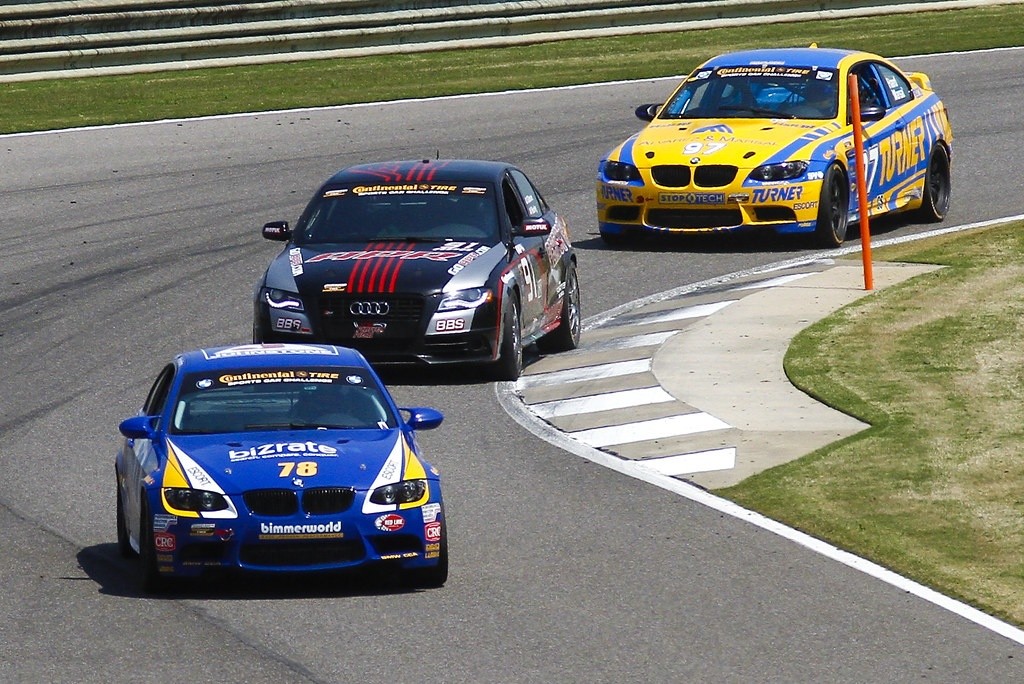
[251,158,582,383]
[595,42,956,250]
[114,343,451,597]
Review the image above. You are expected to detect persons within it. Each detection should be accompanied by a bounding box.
[804,77,835,115]
[303,386,350,419]
